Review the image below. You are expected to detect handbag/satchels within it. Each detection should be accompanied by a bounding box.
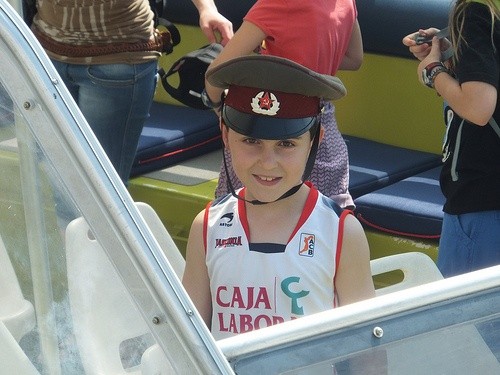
[156,41,224,112]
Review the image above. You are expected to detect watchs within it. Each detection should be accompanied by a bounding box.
[421,62,443,87]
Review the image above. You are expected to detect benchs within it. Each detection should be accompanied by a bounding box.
[0,0,445,300]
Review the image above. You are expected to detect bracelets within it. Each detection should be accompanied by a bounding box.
[424,67,451,88]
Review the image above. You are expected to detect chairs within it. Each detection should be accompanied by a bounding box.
[0,200,500,375]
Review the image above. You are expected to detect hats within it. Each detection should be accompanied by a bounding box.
[205,54,347,140]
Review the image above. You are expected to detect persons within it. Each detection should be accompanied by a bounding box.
[29,0,234,222]
[204,0,362,212]
[181,56,376,341]
[402,0,500,279]
